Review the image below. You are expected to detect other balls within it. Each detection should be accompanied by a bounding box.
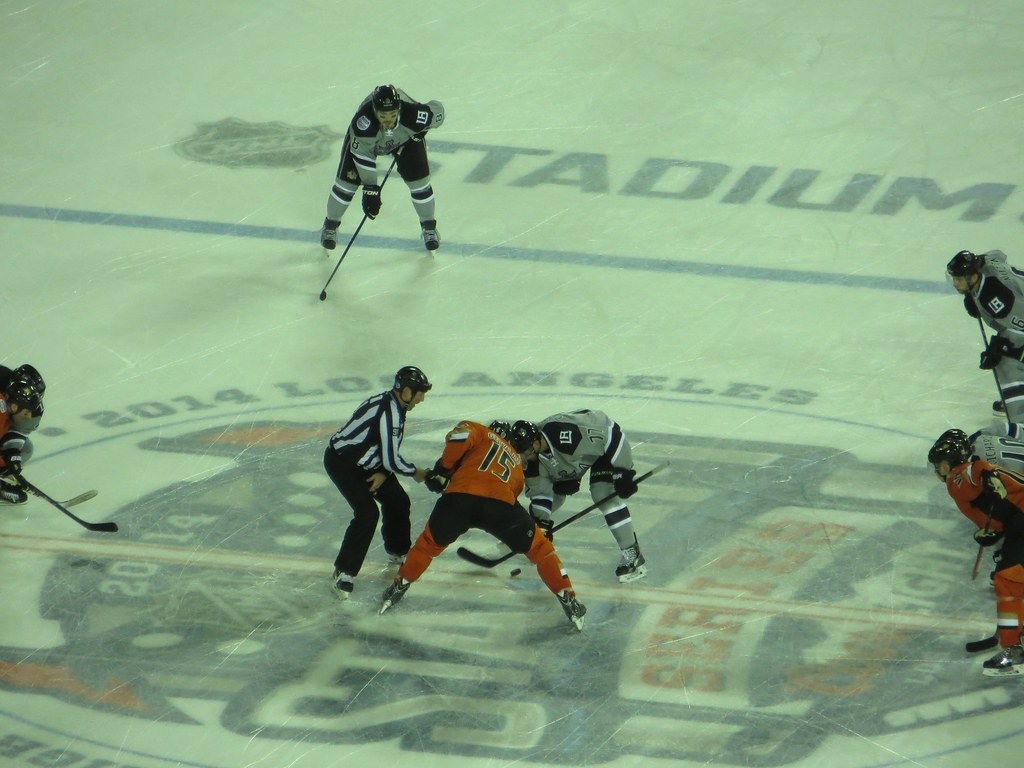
[510,568,521,576]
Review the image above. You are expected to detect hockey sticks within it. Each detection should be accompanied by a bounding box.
[457,460,671,569]
[8,474,98,509]
[16,474,119,532]
[320,145,405,301]
[965,623,1000,652]
[972,504,994,581]
[976,314,1012,424]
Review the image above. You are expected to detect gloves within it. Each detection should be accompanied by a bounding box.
[532,516,554,543]
[613,467,638,499]
[362,184,382,220]
[405,130,429,147]
[1,447,21,475]
[424,458,453,494]
[973,529,1003,547]
[979,335,1009,369]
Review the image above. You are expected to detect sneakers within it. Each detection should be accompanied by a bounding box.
[614,530,647,582]
[0,480,28,505]
[381,575,410,614]
[982,634,1024,676]
[389,551,407,564]
[556,589,587,630]
[989,570,996,587]
[320,217,341,257]
[333,568,354,601]
[420,219,440,259]
[992,399,1006,418]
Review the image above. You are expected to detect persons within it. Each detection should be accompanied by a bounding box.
[510,408,645,576]
[0,364,46,503]
[323,365,433,592]
[929,421,1024,667]
[382,423,587,620]
[947,248,1024,414]
[321,85,445,250]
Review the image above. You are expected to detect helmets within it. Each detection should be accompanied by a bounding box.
[510,420,539,453]
[11,364,46,392]
[393,365,432,399]
[488,420,511,442]
[7,380,44,418]
[947,250,979,276]
[928,428,973,483]
[371,85,400,114]
